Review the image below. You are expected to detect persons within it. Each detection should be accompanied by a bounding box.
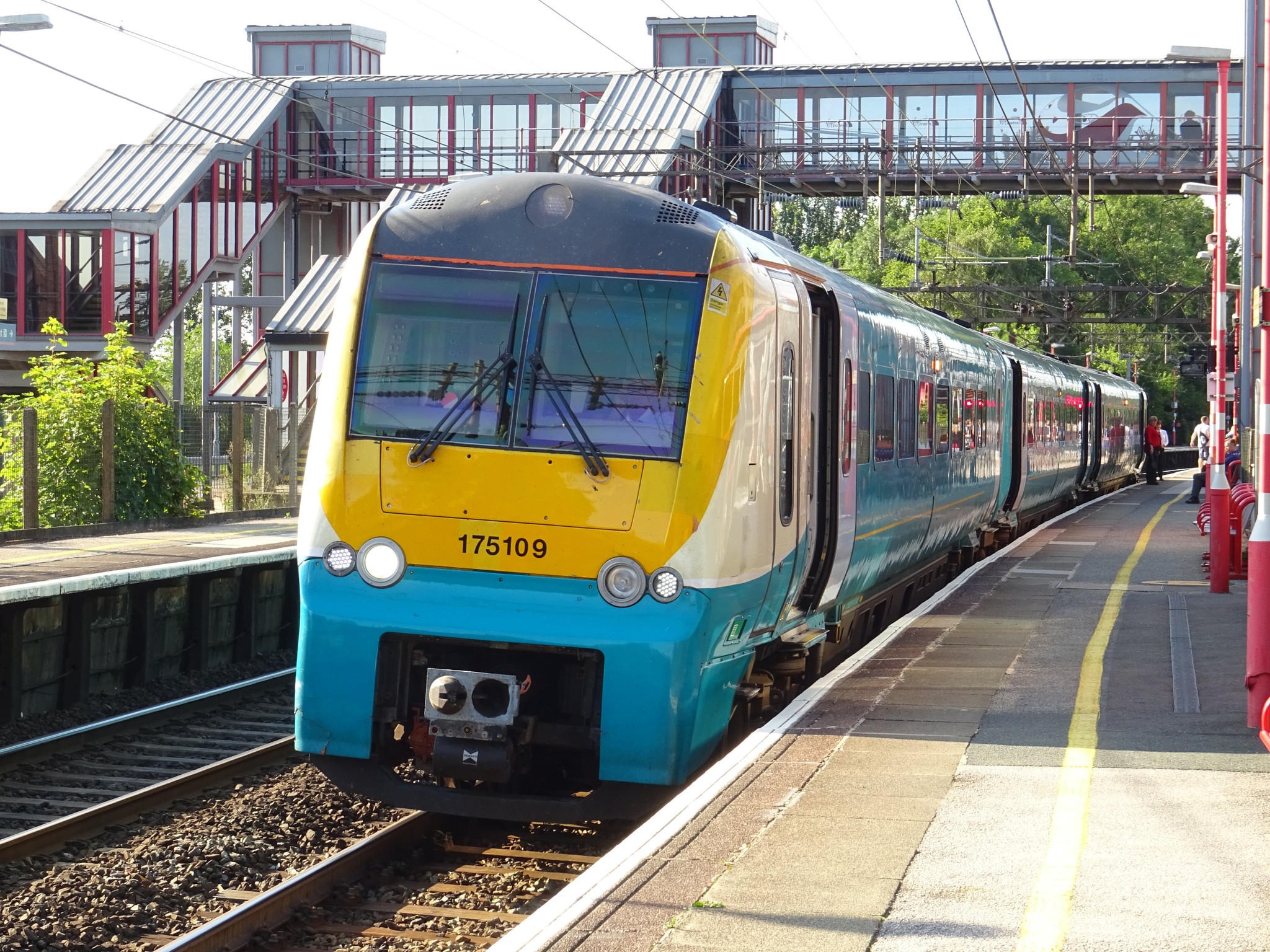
[1202,435,1240,473]
[1145,420,1169,481]
[1102,417,1137,474]
[1144,416,1162,485]
[1180,111,1204,169]
[1189,416,1210,473]
[1026,419,1065,443]
[1185,439,1242,504]
[937,418,983,453]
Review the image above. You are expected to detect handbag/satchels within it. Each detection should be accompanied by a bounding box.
[1199,431,1209,447]
[1144,443,1150,455]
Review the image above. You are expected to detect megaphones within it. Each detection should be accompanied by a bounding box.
[1231,314,1240,324]
[1206,232,1218,245]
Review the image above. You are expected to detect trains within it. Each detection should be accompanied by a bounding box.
[293,169,1148,824]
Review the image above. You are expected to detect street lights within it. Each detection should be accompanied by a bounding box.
[1050,343,1065,355]
[1172,366,1180,446]
[1085,352,1098,369]
[1163,47,1233,589]
[1134,359,1145,383]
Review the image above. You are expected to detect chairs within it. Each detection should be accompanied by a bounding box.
[1196,460,1256,579]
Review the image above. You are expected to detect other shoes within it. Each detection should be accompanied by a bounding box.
[1147,481,1159,485]
[1185,496,1200,504]
